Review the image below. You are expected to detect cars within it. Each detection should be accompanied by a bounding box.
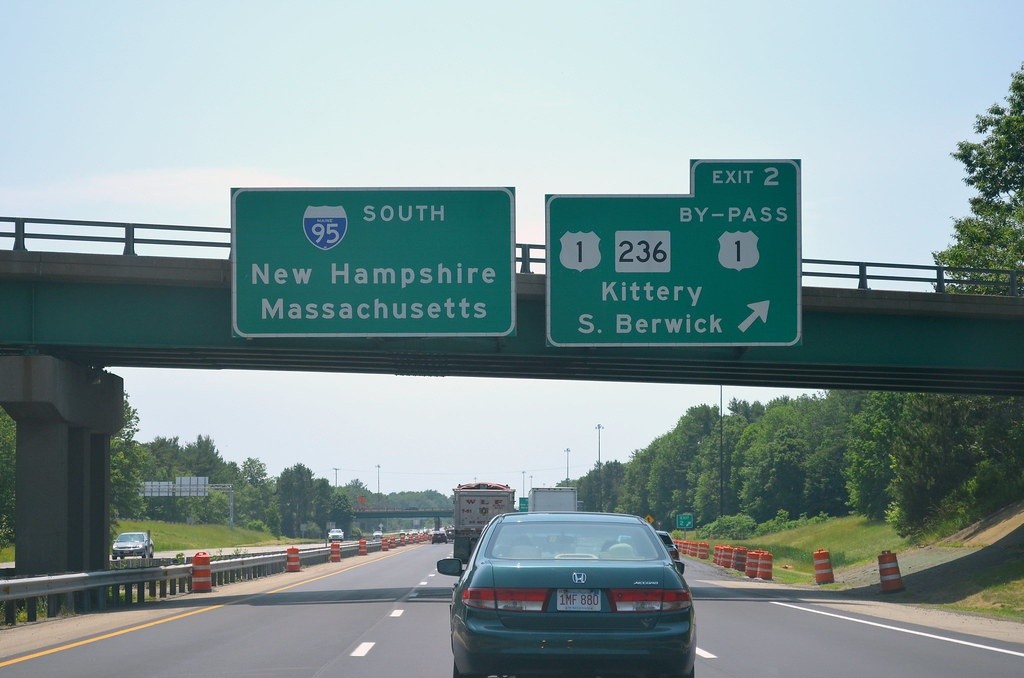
[372,531,383,539]
[111,531,153,559]
[328,528,344,541]
[429,527,455,544]
[414,511,701,677]
[655,528,686,578]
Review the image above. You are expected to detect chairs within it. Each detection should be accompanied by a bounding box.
[504,543,636,558]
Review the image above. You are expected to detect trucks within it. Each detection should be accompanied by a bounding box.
[529,487,578,518]
[451,480,514,563]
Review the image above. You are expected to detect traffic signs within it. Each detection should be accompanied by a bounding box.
[539,158,801,347]
[676,514,694,529]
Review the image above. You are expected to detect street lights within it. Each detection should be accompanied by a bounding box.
[333,467,340,487]
[520,469,533,498]
[595,424,604,511]
[375,464,381,492]
[563,448,572,486]
